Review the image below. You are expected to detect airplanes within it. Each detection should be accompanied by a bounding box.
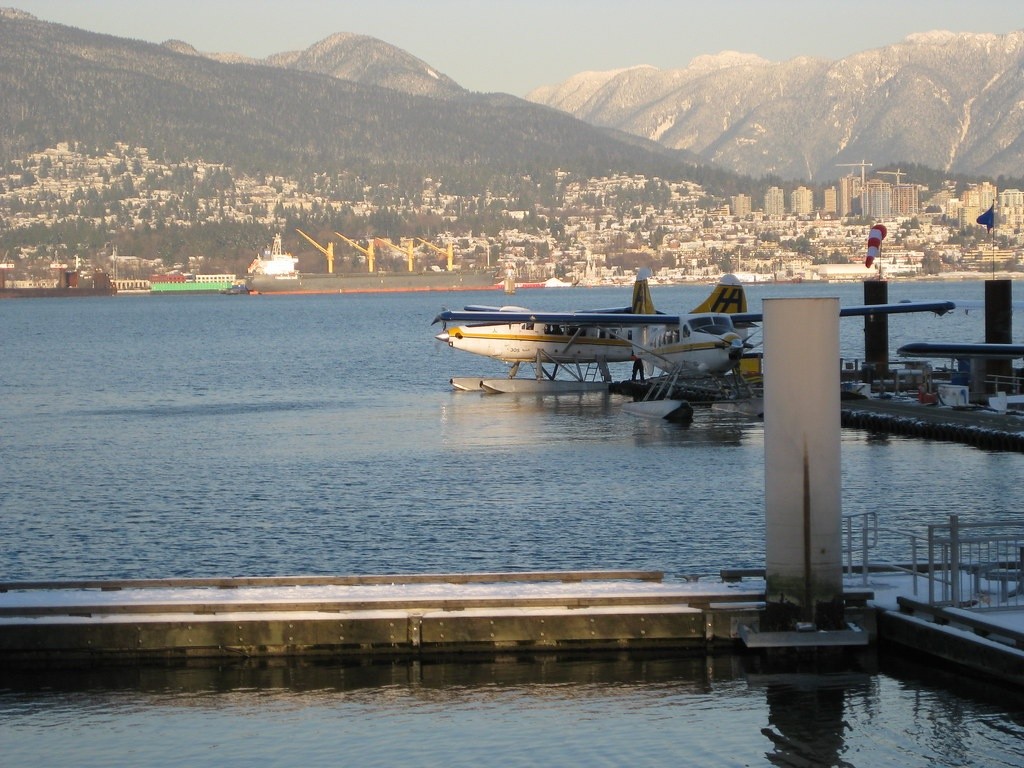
[431,273,957,425]
[427,266,764,398]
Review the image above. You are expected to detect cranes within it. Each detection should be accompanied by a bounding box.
[375,236,414,272]
[877,168,906,185]
[334,231,376,272]
[296,228,335,274]
[836,159,873,185]
[416,237,453,272]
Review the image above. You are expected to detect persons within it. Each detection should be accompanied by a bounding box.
[632,351,645,382]
[546,325,576,336]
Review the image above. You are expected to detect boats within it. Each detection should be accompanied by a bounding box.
[220,283,251,295]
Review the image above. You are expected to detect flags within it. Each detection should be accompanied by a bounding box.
[976,205,994,234]
